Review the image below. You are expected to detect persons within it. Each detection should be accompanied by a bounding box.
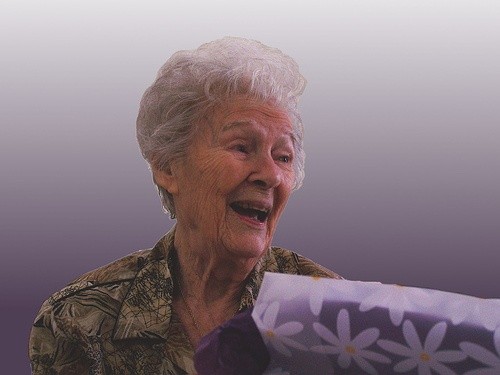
[25,35,347,375]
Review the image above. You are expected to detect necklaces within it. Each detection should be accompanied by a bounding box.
[174,273,244,338]
